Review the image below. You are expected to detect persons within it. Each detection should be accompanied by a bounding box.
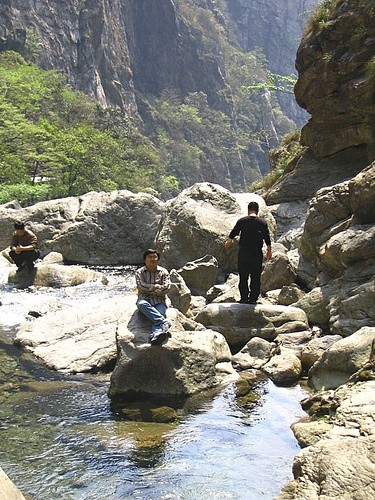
[224,201,272,304]
[135,248,172,344]
[8,221,40,274]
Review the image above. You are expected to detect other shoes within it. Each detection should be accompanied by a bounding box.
[150,332,168,346]
[16,262,27,273]
[162,321,172,333]
[28,262,34,274]
